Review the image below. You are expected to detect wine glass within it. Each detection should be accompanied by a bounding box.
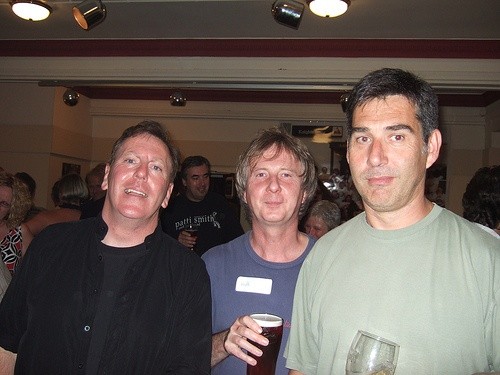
[185,223,198,250]
[346,330,400,375]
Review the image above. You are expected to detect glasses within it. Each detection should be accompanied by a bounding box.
[0,201,10,208]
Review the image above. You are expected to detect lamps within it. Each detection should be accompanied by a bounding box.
[169,92,188,106]
[71,0,106,29]
[11,0,54,22]
[271,0,304,29]
[309,0,350,18]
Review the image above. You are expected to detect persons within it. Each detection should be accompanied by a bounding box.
[305,200,341,240]
[283,67,499,375]
[426,180,447,207]
[0,162,107,304]
[200,128,317,375]
[310,178,332,206]
[0,120,212,375]
[318,166,364,222]
[161,155,224,257]
[462,165,500,233]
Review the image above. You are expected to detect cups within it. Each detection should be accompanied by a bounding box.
[247,314,284,375]
